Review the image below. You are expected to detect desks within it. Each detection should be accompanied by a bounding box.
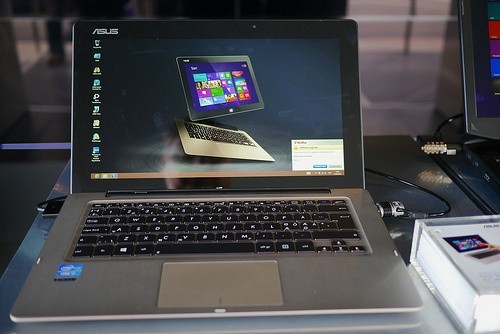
[0,126,500,334]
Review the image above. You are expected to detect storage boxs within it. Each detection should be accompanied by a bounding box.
[408,215,500,334]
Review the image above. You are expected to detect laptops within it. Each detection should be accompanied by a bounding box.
[441,233,499,264]
[10,18,424,322]
[457,0,500,141]
